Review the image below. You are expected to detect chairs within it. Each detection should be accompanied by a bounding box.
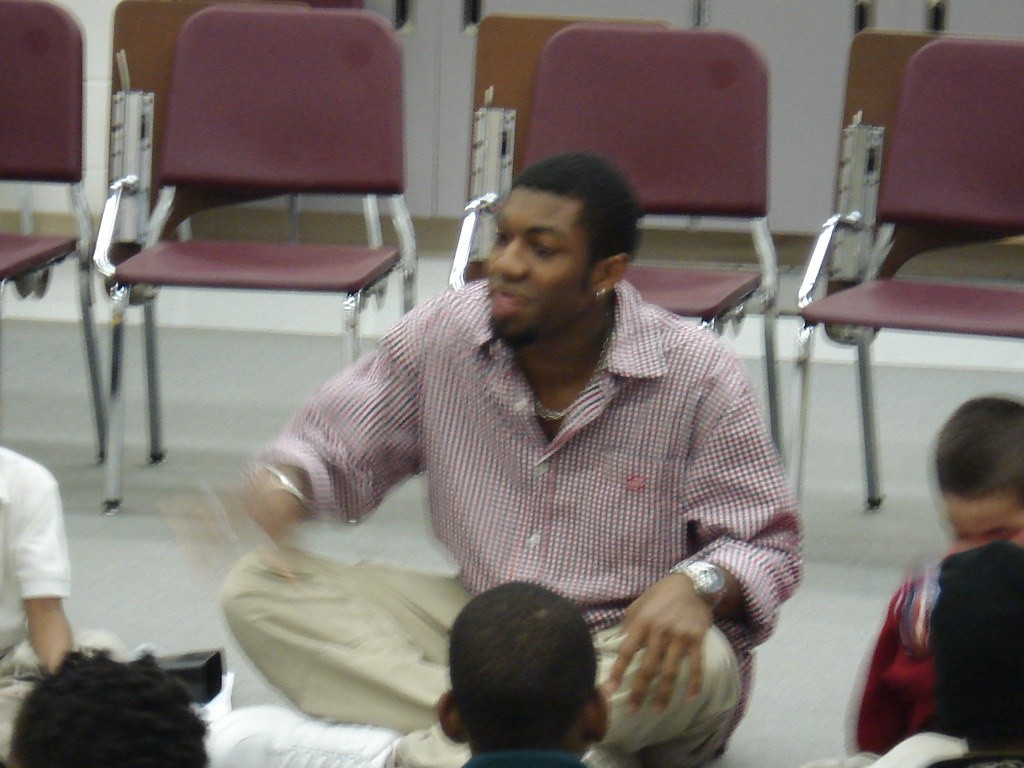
[0,0,107,462]
[525,24,783,456]
[795,40,1024,510]
[93,6,419,517]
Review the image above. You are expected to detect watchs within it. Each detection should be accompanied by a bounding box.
[669,560,727,599]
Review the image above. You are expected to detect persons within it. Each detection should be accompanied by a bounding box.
[0,444,75,767]
[435,581,608,768]
[201,146,802,768]
[847,394,1024,768]
[9,651,209,768]
